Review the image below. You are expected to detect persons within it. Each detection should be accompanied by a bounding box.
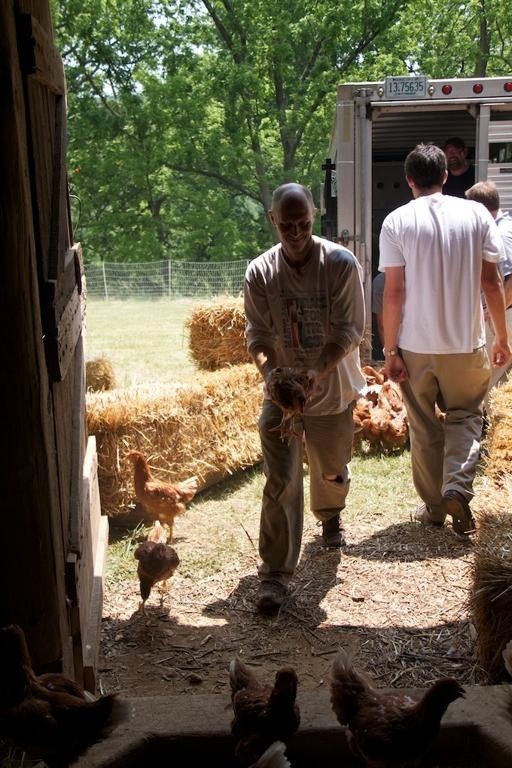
[238,180,366,609]
[378,140,512,534]
[460,179,512,397]
[440,135,476,198]
[373,273,385,346]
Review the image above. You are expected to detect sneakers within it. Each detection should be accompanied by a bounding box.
[425,506,446,527]
[322,513,345,547]
[441,489,476,535]
[258,578,285,604]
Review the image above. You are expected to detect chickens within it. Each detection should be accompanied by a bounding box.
[265,365,317,448]
[301,365,447,476]
[224,653,300,768]
[0,623,131,768]
[133,520,180,617]
[122,449,198,545]
[329,645,468,768]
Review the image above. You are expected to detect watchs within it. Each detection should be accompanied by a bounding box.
[383,347,398,357]
